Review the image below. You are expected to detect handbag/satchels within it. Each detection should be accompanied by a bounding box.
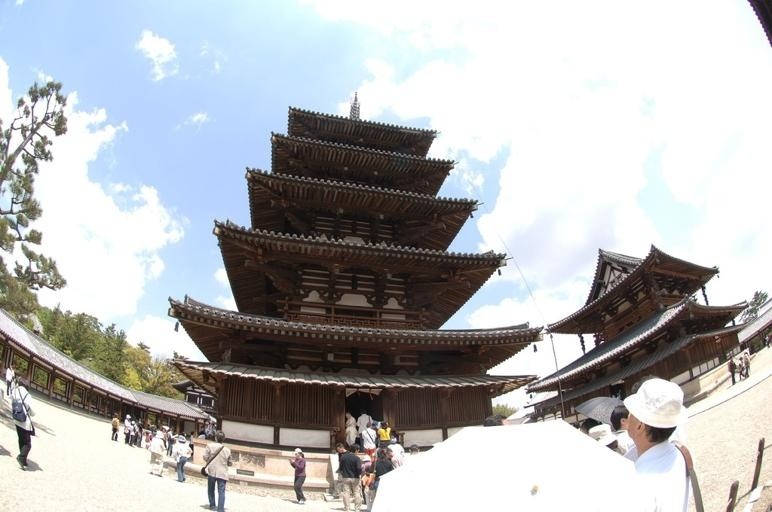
[30,425,36,437]
[201,467,208,477]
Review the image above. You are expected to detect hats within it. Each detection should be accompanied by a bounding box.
[178,435,187,443]
[155,431,166,439]
[291,447,302,455]
[126,413,136,425]
[624,377,689,429]
[588,424,618,446]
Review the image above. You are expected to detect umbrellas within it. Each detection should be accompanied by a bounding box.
[575,394,629,426]
[370,416,665,511]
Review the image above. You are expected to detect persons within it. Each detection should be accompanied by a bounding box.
[587,422,628,457]
[288,446,308,504]
[6,363,16,396]
[331,411,406,512]
[11,377,36,470]
[579,417,601,434]
[202,431,231,512]
[148,431,167,477]
[11,375,17,398]
[608,404,636,453]
[110,412,217,461]
[726,350,750,387]
[482,414,513,427]
[616,375,694,512]
[172,434,193,482]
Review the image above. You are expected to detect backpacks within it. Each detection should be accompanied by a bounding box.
[11,392,30,422]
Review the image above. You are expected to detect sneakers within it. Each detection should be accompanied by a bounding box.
[296,497,307,504]
[150,471,187,483]
[17,456,28,470]
